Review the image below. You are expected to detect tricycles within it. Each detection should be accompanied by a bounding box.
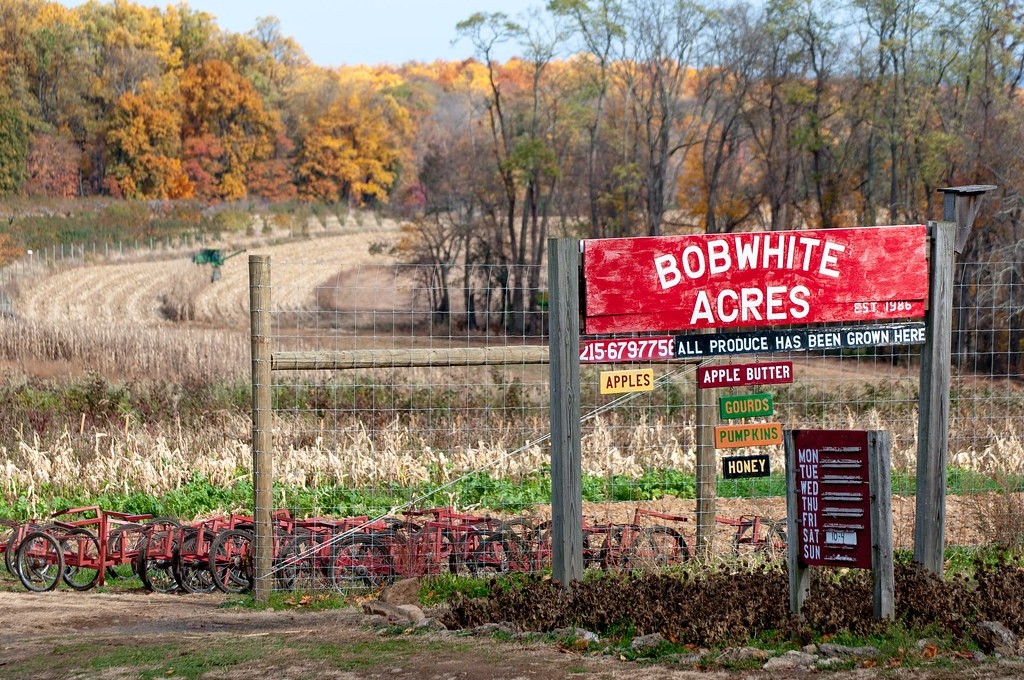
[0,499,789,595]
